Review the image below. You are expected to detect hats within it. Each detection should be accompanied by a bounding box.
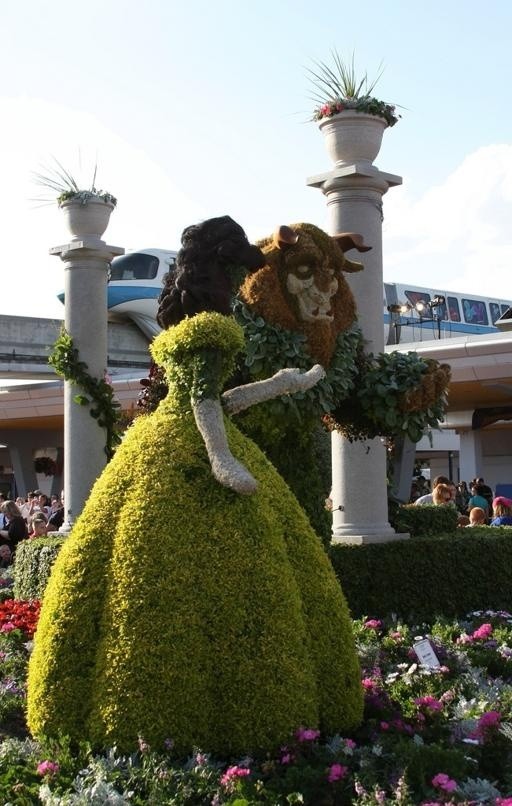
[31,512,48,523]
[491,496,510,509]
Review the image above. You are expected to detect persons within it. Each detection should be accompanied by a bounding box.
[407,465,511,528]
[1,488,64,571]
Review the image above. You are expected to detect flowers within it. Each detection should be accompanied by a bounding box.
[290,42,410,127]
[25,143,116,207]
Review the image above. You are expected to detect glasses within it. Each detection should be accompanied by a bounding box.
[445,497,450,502]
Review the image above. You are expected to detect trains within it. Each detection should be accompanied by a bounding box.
[56,248,512,343]
